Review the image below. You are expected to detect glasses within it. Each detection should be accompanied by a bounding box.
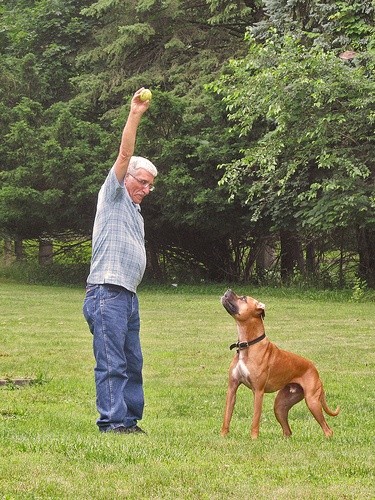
[128,173,155,191]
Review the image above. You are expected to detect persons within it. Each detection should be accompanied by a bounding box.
[83,88,157,434]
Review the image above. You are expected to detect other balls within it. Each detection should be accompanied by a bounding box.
[140,88,153,102]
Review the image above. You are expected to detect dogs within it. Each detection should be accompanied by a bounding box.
[221,289,340,442]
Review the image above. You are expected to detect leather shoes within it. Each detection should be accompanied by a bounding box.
[129,426,144,434]
[114,427,128,434]
